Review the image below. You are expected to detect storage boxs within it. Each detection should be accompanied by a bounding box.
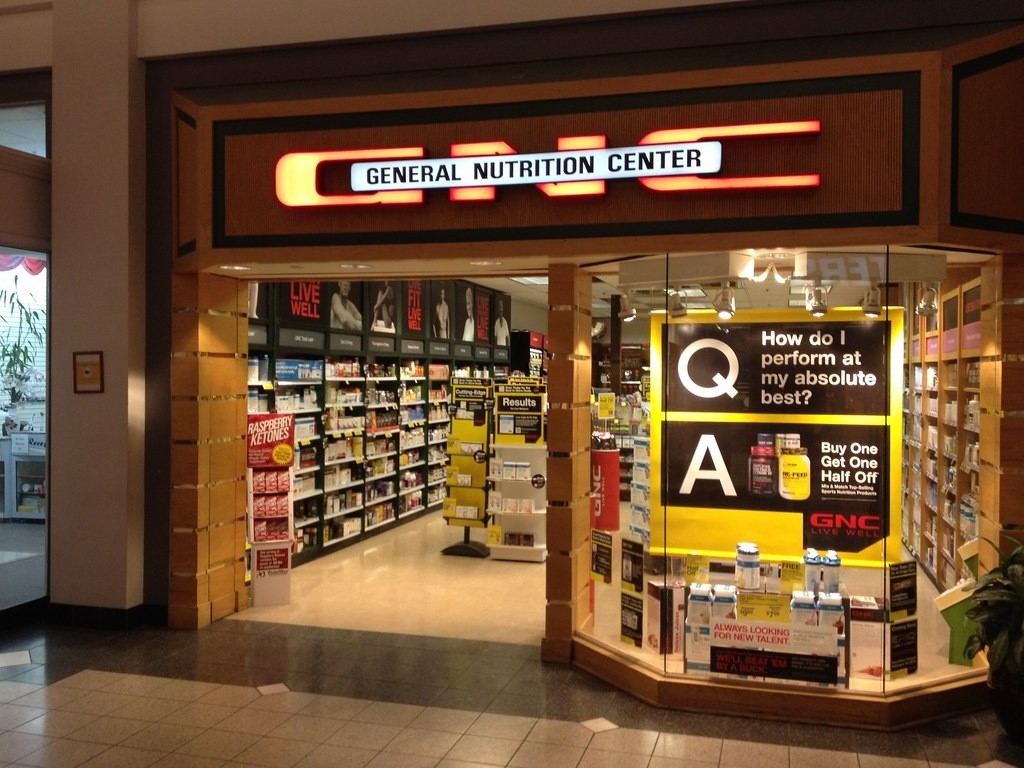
[610,419,651,543]
[644,542,843,672]
[489,463,535,547]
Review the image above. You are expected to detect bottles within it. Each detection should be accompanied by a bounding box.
[803,548,841,595]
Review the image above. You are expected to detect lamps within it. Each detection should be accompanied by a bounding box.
[668,284,687,317]
[914,281,937,318]
[618,295,637,321]
[803,279,828,317]
[862,284,882,318]
[713,281,736,321]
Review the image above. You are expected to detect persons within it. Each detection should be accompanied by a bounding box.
[431,283,451,339]
[330,279,362,330]
[370,278,396,332]
[492,297,511,346]
[461,287,475,341]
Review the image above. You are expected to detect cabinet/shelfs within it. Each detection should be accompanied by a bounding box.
[617,448,635,493]
[489,444,549,562]
[901,276,983,593]
[682,584,850,690]
[247,316,509,579]
[0,431,48,521]
